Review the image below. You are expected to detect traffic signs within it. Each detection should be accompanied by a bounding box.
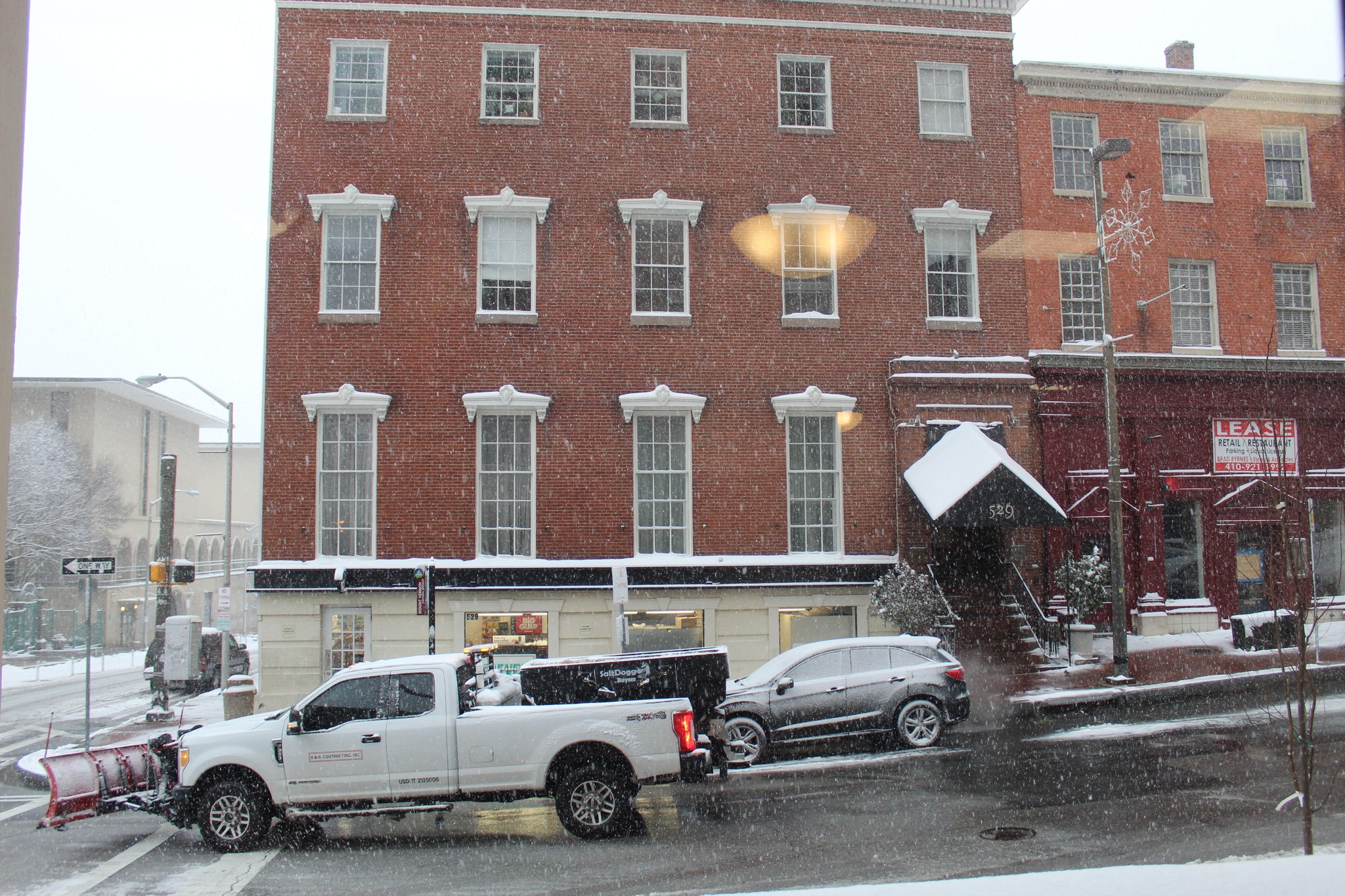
[58,556,116,576]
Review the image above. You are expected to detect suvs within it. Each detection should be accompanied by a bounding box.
[718,636,972,769]
[141,626,252,693]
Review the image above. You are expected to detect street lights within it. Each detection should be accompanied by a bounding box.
[135,374,235,689]
[1089,135,1134,686]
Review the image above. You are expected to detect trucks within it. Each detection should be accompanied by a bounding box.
[461,642,727,777]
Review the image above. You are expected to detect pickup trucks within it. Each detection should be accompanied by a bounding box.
[31,654,696,843]
